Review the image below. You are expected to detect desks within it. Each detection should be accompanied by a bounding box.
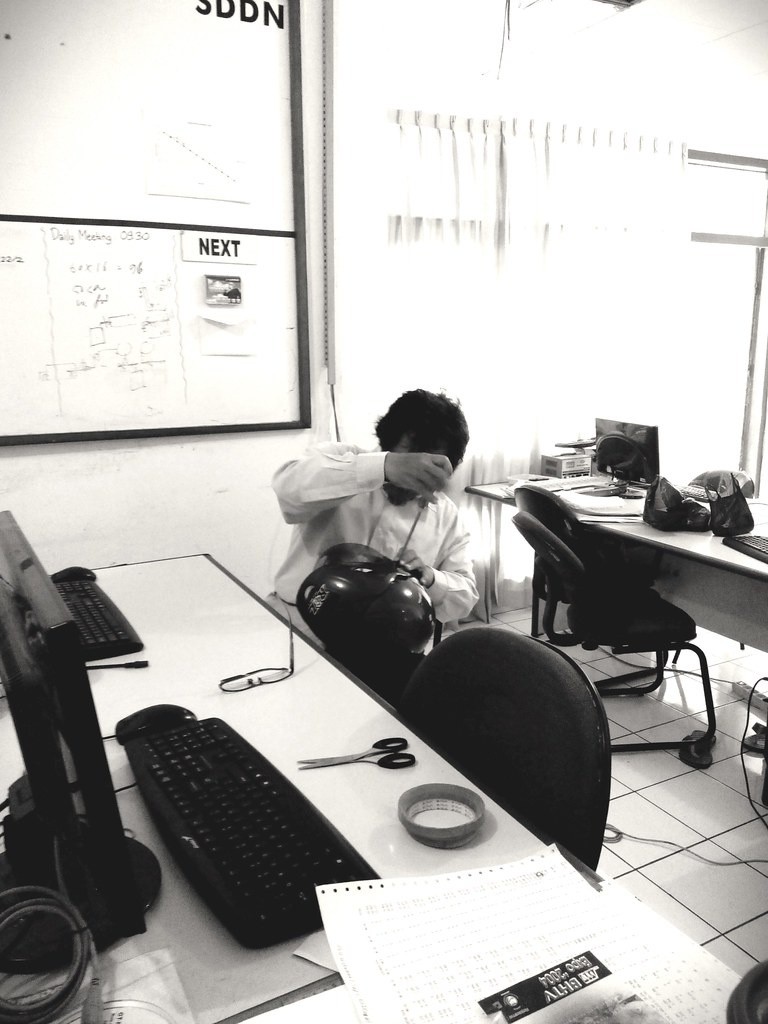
[0,556,741,1024]
[465,474,767,659]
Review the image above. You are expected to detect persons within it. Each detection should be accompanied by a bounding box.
[269,388,480,710]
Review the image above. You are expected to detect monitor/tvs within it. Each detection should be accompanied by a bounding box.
[595,419,659,499]
[0,510,164,974]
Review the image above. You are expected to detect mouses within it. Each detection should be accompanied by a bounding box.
[51,566,96,584]
[115,704,197,745]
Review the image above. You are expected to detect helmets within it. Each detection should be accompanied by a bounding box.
[296,542,436,670]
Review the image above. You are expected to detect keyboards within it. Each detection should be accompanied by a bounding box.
[52,580,144,661]
[125,718,388,950]
[678,485,718,503]
[722,535,768,564]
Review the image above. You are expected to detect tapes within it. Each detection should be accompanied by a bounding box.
[397,782,486,850]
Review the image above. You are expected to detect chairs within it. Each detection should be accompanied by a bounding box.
[505,482,716,754]
[403,627,614,883]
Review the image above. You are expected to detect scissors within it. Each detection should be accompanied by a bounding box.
[295,737,415,770]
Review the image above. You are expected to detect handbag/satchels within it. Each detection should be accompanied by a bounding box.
[643,473,711,533]
[705,473,754,538]
